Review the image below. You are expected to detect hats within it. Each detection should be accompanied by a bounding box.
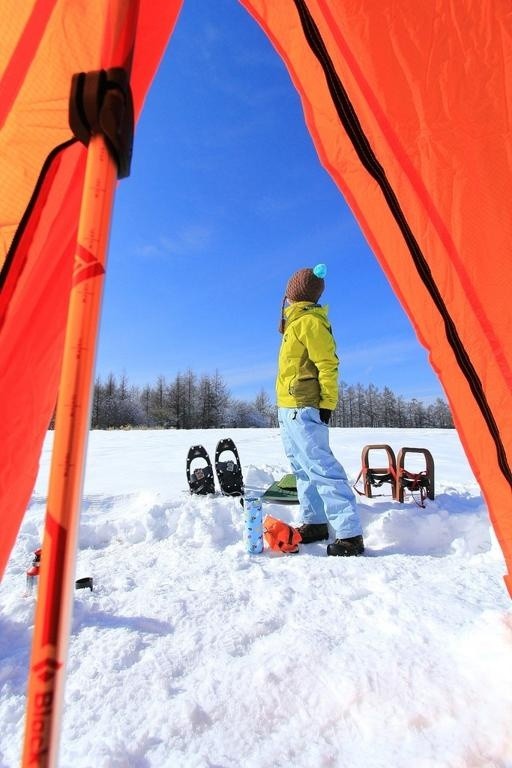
[279,263,327,333]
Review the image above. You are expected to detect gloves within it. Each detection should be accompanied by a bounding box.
[319,408,331,424]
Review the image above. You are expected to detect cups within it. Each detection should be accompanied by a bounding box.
[75,577,94,592]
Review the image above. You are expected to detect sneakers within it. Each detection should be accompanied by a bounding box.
[327,535,364,556]
[298,524,329,544]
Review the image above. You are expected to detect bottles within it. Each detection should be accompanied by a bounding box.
[243,496,264,556]
[26,548,40,596]
[404,486,409,504]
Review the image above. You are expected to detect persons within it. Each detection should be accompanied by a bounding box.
[275,264,365,555]
